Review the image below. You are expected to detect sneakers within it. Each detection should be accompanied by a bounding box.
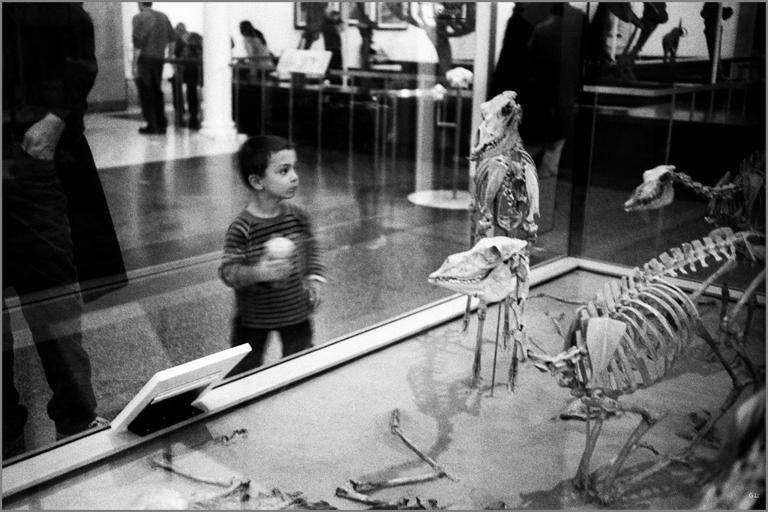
[138,114,201,134]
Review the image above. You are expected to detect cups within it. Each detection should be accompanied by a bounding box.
[263,238,296,289]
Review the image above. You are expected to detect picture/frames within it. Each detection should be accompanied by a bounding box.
[294,2,409,30]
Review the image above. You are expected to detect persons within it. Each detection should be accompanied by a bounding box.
[131,1,177,136]
[305,6,342,87]
[239,21,276,76]
[218,134,326,377]
[1,1,131,460]
[488,1,602,236]
[169,21,204,132]
